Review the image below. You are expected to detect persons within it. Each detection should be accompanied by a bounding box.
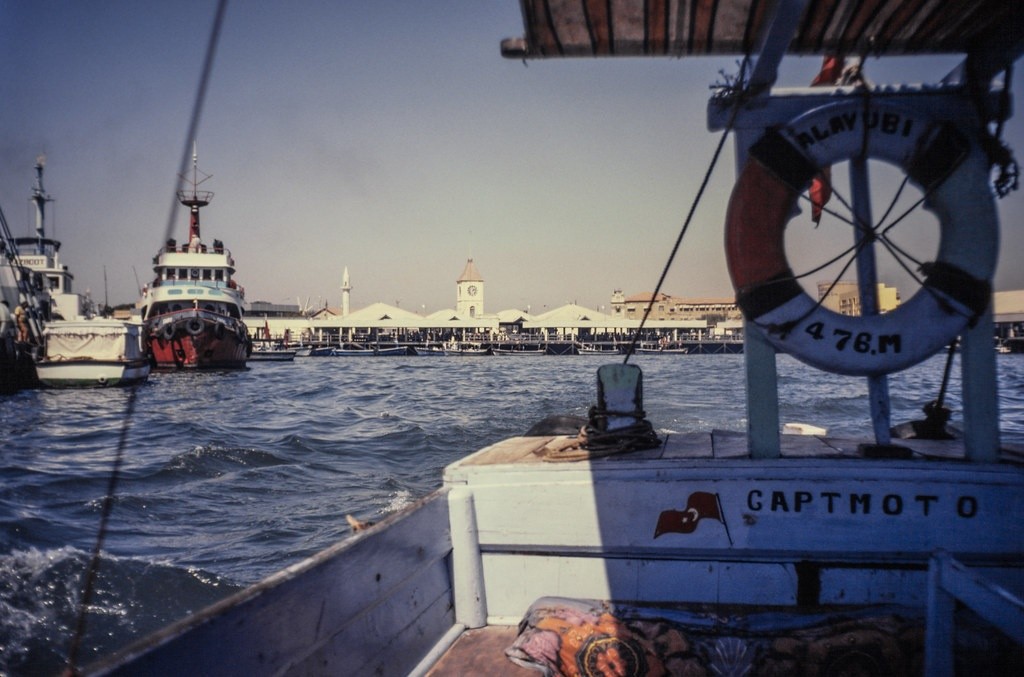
[253,332,257,340]
[584,331,660,341]
[189,234,201,253]
[352,333,355,342]
[389,330,463,342]
[470,331,579,341]
[15,301,30,343]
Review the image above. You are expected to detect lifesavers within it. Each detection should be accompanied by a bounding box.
[163,321,177,340]
[723,95,1001,374]
[235,327,245,341]
[186,316,204,334]
[214,321,227,340]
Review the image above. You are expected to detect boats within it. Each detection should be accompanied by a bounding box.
[0,156,153,395]
[577,336,619,356]
[635,337,691,355]
[295,339,550,358]
[137,137,254,375]
[249,312,298,361]
